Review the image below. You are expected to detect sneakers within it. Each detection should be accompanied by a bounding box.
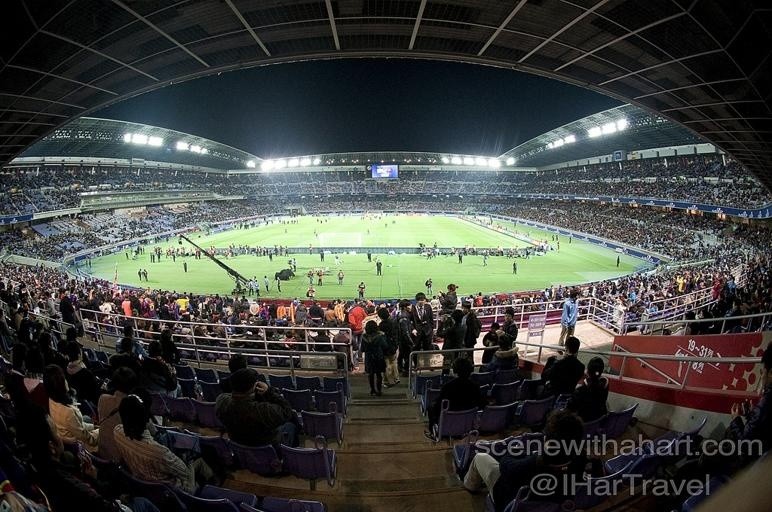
[423,431,441,445]
[383,377,402,389]
[367,388,384,398]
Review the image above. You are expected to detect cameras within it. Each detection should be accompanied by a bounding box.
[741,401,751,415]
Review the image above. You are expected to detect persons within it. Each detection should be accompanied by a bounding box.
[1,154,771,510]
